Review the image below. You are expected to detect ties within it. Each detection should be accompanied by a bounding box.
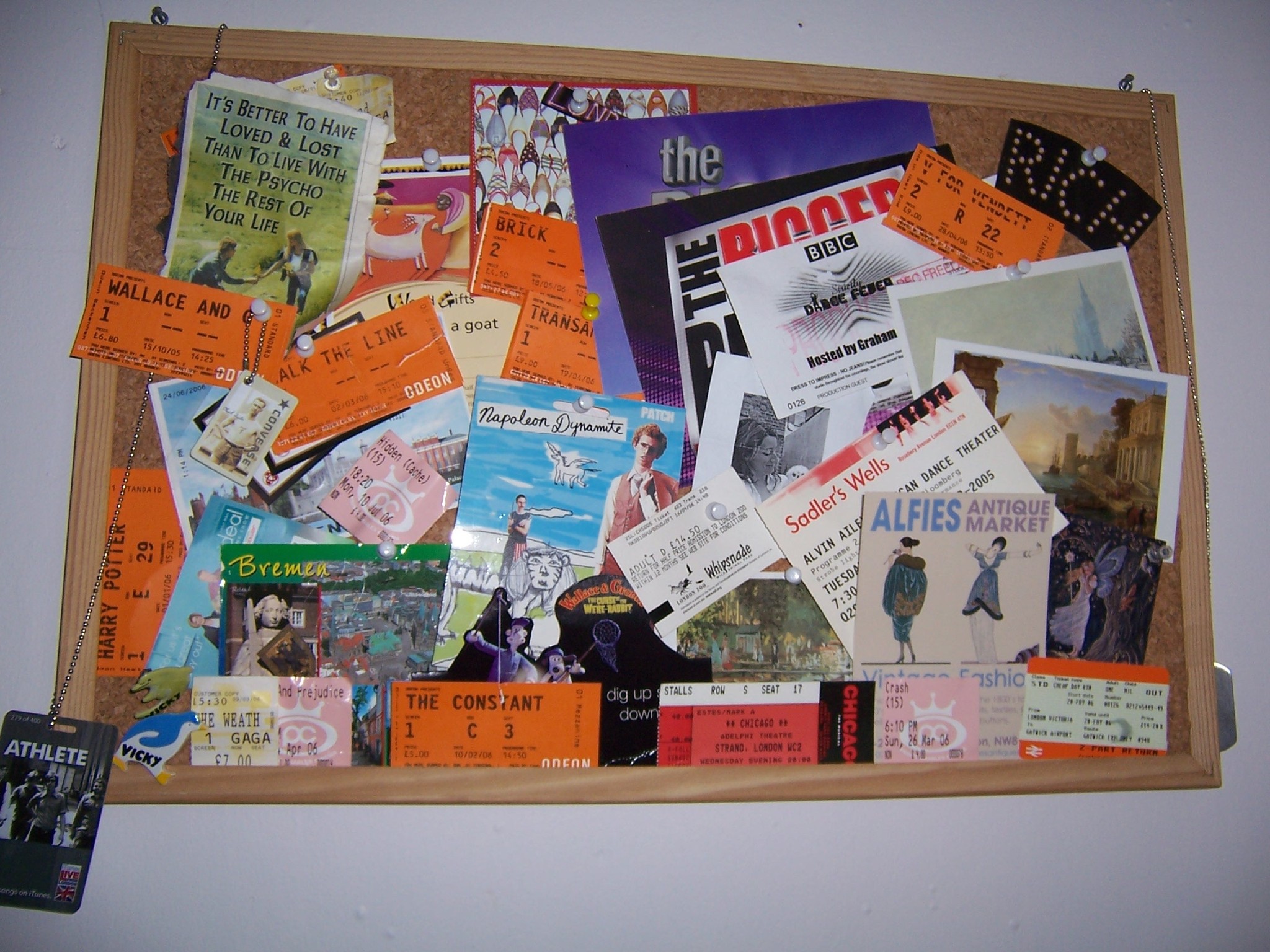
[635,478,643,489]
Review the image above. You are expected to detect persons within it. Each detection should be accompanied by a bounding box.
[591,423,679,575]
[188,610,220,649]
[503,494,531,573]
[231,594,291,677]
[731,418,787,505]
[257,231,319,317]
[196,569,220,609]
[209,398,265,474]
[188,236,255,291]
